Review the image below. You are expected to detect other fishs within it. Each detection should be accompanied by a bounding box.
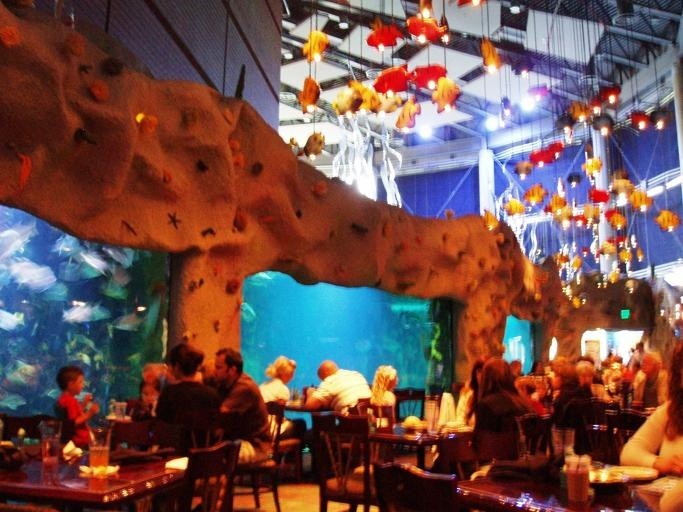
[0,216,145,411]
[249,270,272,284]
[237,300,257,324]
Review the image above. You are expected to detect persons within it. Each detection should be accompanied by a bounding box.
[258,355,398,477]
[56,342,271,476]
[458,340,683,477]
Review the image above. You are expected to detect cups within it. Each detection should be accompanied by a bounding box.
[114,401,127,423]
[425,399,441,435]
[564,457,593,502]
[39,420,62,472]
[88,426,111,478]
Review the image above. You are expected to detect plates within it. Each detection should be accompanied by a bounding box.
[590,466,661,487]
